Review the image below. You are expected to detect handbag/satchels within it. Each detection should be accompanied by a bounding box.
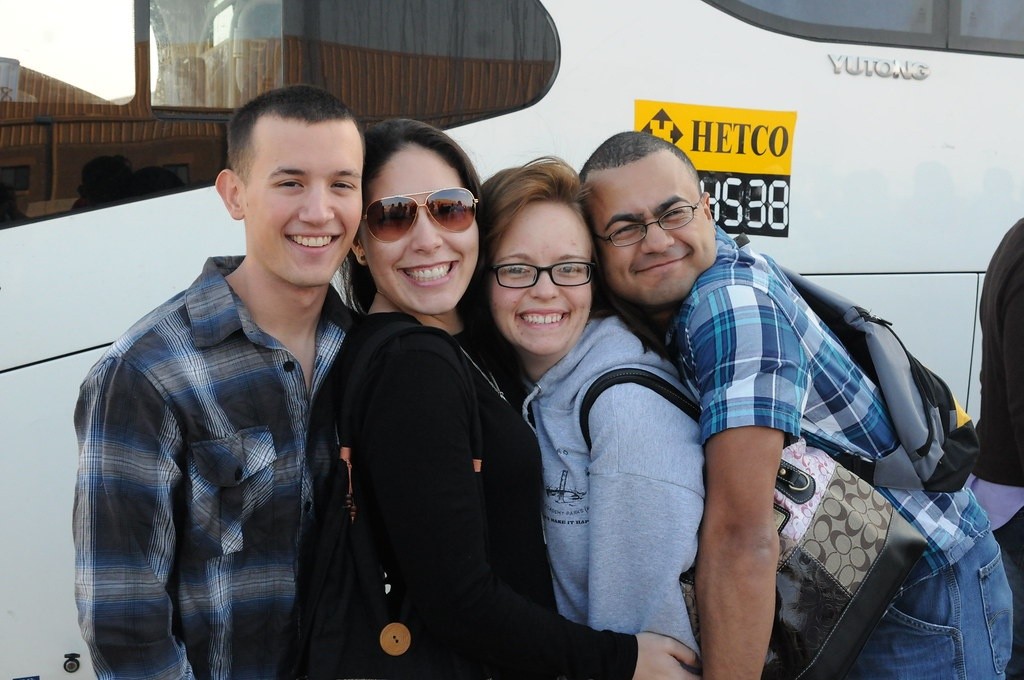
[580,368,929,680]
[284,321,504,680]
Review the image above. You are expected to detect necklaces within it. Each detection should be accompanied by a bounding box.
[456,340,508,401]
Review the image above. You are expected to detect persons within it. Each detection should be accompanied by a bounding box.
[972,220,1024,680]
[73,86,1012,680]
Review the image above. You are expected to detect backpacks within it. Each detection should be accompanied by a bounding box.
[734,233,978,494]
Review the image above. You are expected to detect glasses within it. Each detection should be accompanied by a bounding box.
[592,194,703,247]
[484,261,595,289]
[362,186,479,243]
